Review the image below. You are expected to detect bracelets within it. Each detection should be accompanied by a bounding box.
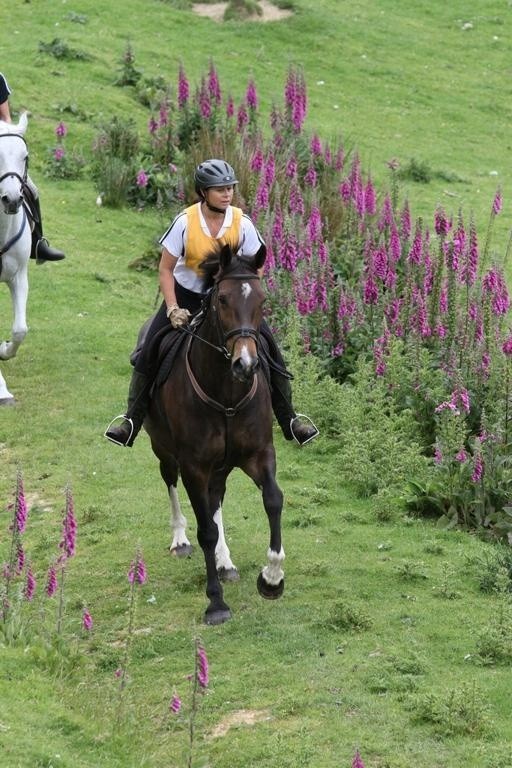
[166,304,179,318]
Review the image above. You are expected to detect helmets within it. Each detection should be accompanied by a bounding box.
[195,158,239,189]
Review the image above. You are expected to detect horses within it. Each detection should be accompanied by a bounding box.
[142,231,286,627]
[0,110,33,408]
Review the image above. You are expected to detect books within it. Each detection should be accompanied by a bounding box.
[0,72,65,261]
[106,159,317,447]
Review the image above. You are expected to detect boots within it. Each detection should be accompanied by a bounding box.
[31,198,65,260]
[107,369,154,448]
[270,373,316,444]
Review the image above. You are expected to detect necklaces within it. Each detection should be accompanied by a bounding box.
[203,203,221,228]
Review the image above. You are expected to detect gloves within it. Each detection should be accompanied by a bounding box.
[166,304,192,329]
